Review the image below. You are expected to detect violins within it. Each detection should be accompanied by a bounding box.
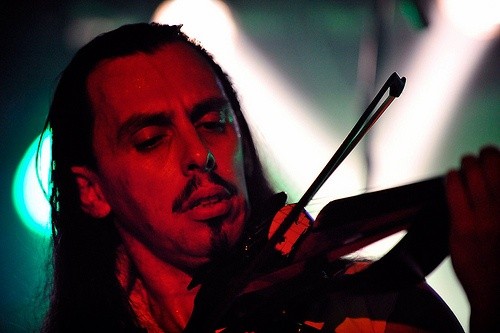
[223,157,463,329]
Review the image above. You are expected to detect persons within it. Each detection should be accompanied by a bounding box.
[41,21,500,333]
